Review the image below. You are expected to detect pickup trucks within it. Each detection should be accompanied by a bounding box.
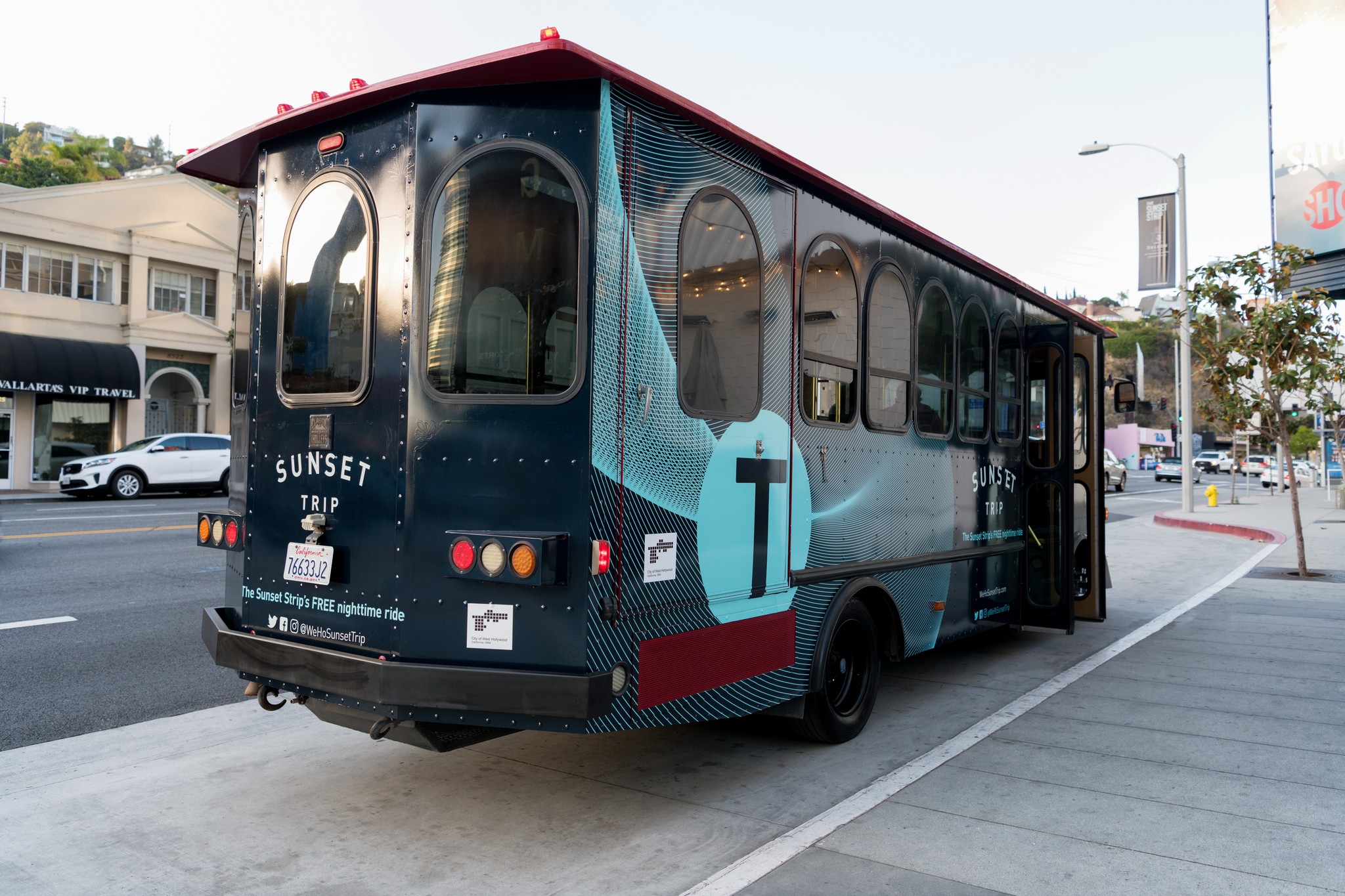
[1193,450,1234,475]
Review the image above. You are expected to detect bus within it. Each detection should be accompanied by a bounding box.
[172,24,1151,756]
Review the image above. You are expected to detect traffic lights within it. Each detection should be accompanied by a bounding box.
[1292,403,1299,417]
[1171,423,1178,442]
[1161,397,1167,411]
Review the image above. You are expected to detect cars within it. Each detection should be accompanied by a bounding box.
[1240,455,1277,477]
[1260,457,1322,489]
[57,432,232,500]
[1234,461,1240,473]
[1155,457,1204,482]
[1101,448,1130,491]
[1139,458,1159,470]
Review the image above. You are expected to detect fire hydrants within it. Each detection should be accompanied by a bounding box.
[1205,485,1219,507]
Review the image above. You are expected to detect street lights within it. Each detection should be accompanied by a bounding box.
[1078,142,1193,514]
